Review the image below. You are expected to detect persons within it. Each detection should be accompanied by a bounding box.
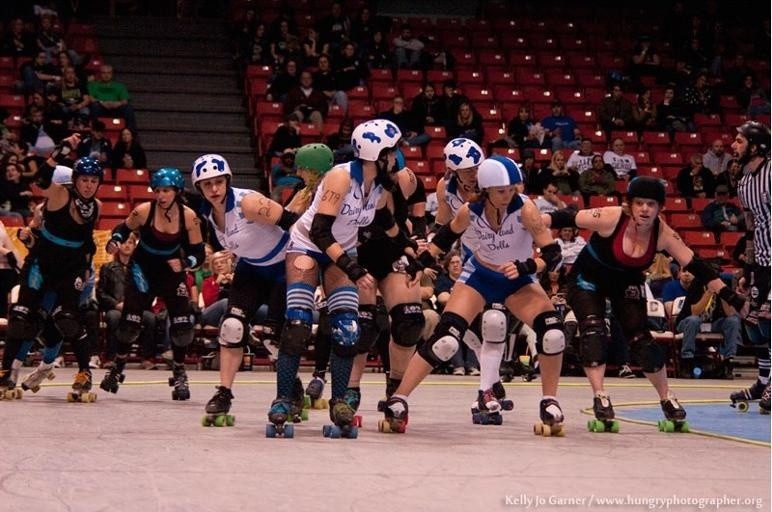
[0,0,771,439]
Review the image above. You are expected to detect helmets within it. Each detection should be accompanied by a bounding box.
[476,154,522,190]
[152,167,185,193]
[294,143,334,172]
[192,154,231,189]
[443,137,483,171]
[72,157,102,186]
[627,176,666,205]
[739,119,770,150]
[350,119,402,161]
